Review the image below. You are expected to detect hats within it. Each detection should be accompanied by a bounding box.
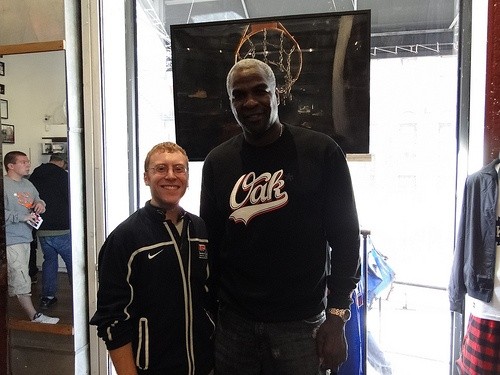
[51,152,68,164]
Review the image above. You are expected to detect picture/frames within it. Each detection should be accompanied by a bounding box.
[0,99,9,119]
[1,123,14,143]
[0,61,5,76]
[0,84,5,94]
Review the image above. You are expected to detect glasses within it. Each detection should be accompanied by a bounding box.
[147,164,188,175]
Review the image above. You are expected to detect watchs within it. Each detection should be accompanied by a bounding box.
[325,306,351,323]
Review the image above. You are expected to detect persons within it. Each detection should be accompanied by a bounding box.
[3,150,61,324]
[449,161,500,375]
[200,58,363,375]
[28,152,73,309]
[89,143,216,375]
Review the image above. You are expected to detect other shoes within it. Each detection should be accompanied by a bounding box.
[30,271,39,284]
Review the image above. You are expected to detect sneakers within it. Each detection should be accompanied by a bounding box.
[31,312,60,324]
[36,295,58,310]
